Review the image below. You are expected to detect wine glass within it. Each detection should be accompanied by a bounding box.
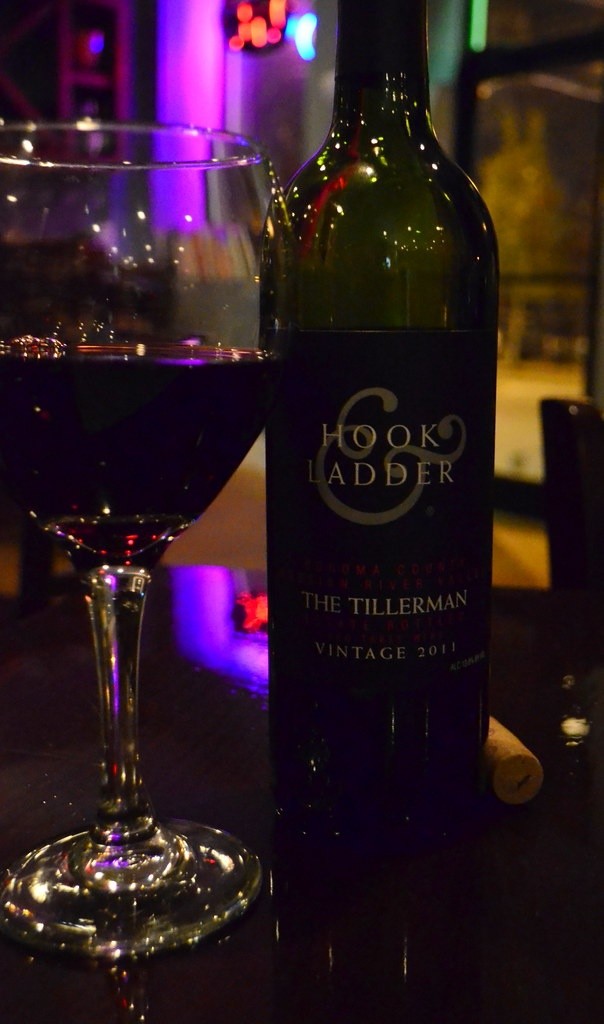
[0,113,273,961]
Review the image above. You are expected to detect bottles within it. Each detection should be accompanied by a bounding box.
[245,1,498,849]
